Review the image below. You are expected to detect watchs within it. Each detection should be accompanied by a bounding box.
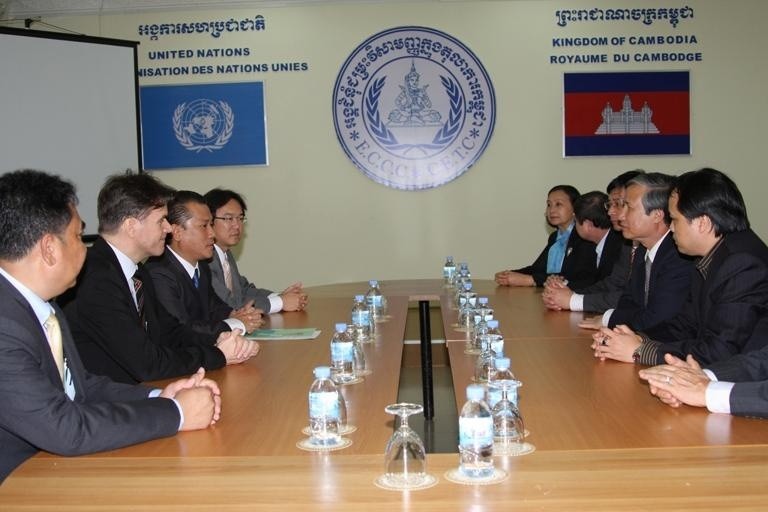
[633,344,644,361]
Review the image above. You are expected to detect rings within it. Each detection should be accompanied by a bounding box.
[666,375,672,384]
[601,337,609,345]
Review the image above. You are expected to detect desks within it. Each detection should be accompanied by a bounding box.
[0,278,768,512]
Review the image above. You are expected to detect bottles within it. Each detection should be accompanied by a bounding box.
[306,365,342,445]
[487,357,516,437]
[454,384,498,477]
[327,278,386,382]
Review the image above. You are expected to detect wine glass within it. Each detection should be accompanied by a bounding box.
[380,401,430,487]
[444,255,516,382]
[489,379,526,454]
[328,367,349,432]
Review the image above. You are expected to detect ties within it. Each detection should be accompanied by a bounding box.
[131,276,146,318]
[44,312,64,382]
[192,267,200,288]
[221,256,234,291]
[629,240,638,260]
[645,257,652,304]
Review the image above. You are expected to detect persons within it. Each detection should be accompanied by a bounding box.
[0,169,223,490]
[495,170,697,334]
[639,344,768,417]
[592,170,768,369]
[143,189,267,336]
[199,186,309,316]
[54,169,262,385]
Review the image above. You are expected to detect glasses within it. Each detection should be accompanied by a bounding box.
[214,215,247,226]
[604,200,623,211]
